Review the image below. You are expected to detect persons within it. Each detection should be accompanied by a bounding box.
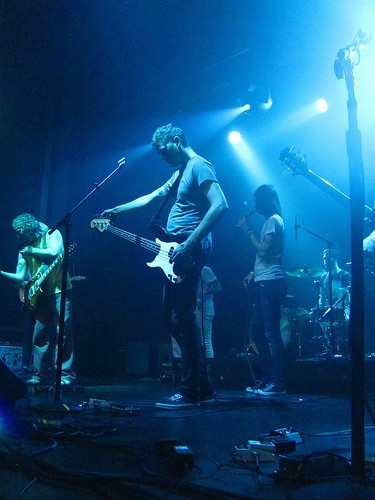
[317,249,351,357]
[172,265,221,386]
[235,184,288,395]
[99,123,229,410]
[363,229,375,252]
[0,213,77,387]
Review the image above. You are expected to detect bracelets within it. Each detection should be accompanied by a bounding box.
[247,230,254,237]
[369,236,375,243]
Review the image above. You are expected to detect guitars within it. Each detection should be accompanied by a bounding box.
[19,242,78,312]
[90,213,202,283]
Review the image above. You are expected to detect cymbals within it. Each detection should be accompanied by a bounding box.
[285,267,326,278]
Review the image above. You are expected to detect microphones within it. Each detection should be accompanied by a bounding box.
[294,216,298,240]
[244,209,254,219]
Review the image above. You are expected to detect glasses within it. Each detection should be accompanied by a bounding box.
[158,138,172,155]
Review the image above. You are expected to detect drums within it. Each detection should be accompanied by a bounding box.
[312,306,345,322]
[248,307,315,358]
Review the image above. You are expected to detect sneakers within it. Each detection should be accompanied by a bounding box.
[246,382,267,392]
[155,389,218,410]
[24,374,45,385]
[254,382,288,395]
[48,376,72,392]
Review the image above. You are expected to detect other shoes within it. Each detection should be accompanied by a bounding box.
[368,352,375,358]
[319,351,333,358]
